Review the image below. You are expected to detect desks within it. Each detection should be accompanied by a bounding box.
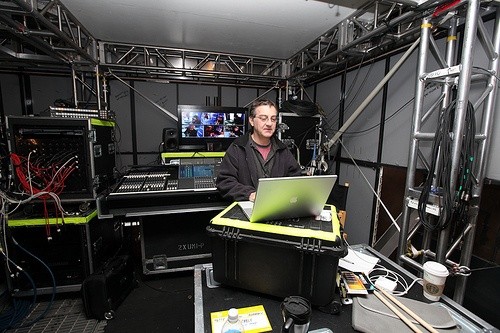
[194,244,500,333]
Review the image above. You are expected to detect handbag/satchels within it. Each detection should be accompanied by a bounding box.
[282,100,318,117]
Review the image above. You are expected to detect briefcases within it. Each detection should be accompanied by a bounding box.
[82,256,139,319]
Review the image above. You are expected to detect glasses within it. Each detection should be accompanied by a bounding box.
[254,115,278,122]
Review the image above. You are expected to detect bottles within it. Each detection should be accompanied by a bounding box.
[220,308,243,333]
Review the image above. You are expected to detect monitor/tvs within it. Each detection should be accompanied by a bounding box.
[177,104,248,152]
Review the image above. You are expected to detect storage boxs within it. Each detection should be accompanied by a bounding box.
[206,201,348,305]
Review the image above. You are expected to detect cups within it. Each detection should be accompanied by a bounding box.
[280,296,312,333]
[423,262,449,301]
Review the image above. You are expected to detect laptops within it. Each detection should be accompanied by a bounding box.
[237,175,337,223]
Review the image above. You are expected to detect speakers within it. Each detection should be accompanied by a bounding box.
[163,128,178,152]
